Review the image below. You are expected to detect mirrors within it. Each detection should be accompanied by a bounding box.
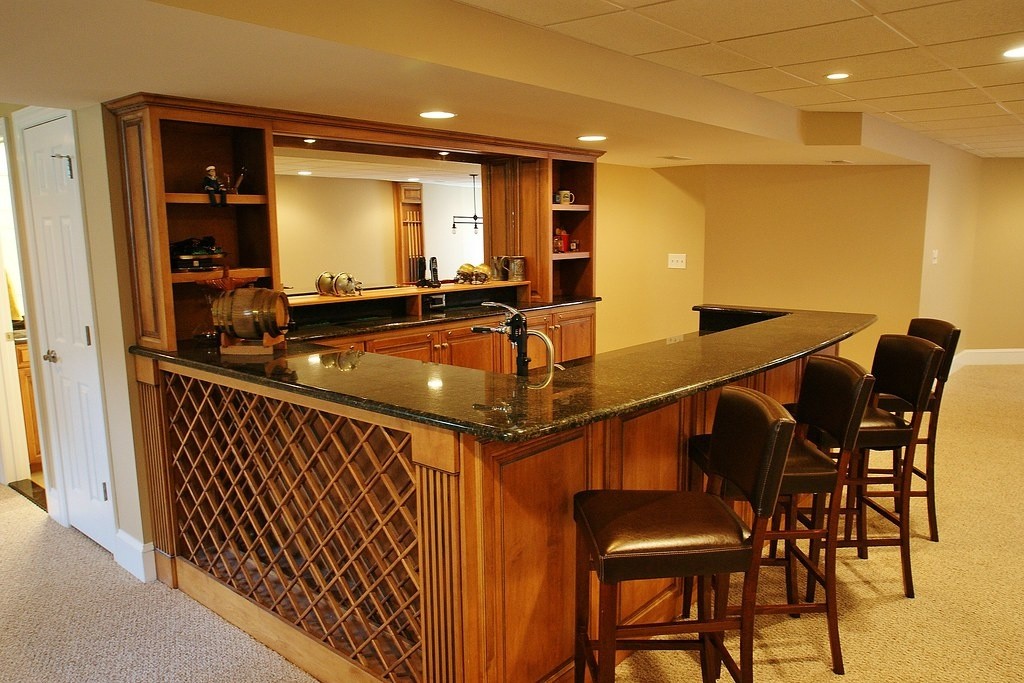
[273,135,515,295]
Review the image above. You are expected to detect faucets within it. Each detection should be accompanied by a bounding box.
[470,302,533,373]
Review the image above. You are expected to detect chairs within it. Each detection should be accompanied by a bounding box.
[573,385,797,683]
[682,355,875,680]
[770,335,946,603]
[830,319,962,542]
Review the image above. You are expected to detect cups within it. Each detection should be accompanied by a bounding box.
[571,240,579,252]
[554,236,564,253]
[552,194,561,204]
[501,256,526,281]
[559,191,575,204]
[490,256,508,281]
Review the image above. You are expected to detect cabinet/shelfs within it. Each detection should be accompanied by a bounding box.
[106,91,608,375]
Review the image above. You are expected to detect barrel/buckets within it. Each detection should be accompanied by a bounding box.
[211,288,290,338]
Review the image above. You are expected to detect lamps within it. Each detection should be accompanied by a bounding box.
[452,174,483,234]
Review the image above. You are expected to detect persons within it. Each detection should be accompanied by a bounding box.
[203,165,229,207]
